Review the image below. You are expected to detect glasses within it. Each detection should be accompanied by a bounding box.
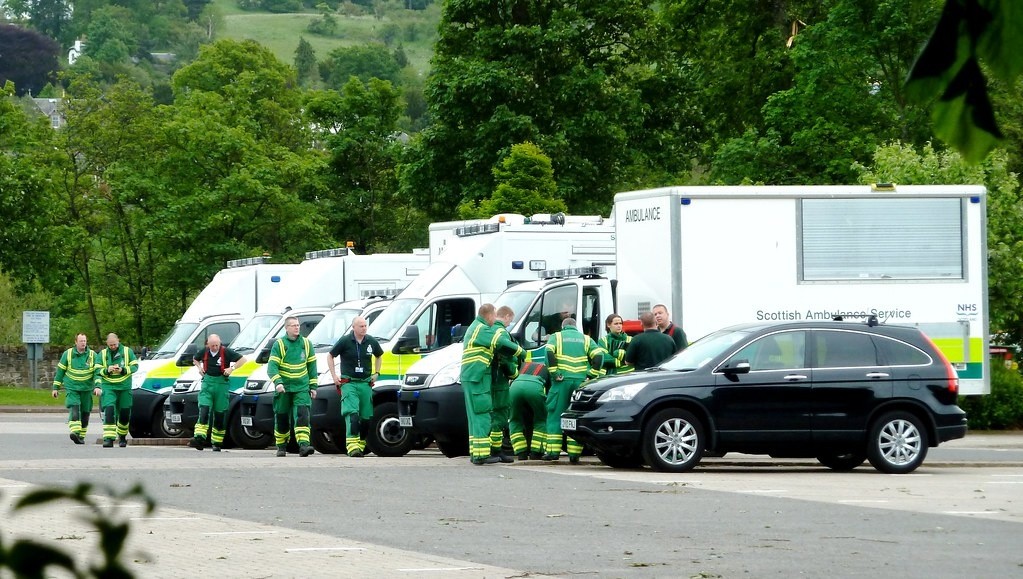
[289,324,300,328]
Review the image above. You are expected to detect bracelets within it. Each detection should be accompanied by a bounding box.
[231,366,235,371]
[376,372,381,375]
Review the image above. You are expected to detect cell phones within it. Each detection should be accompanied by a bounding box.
[114,368,119,369]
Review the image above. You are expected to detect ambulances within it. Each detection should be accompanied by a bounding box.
[395,181,991,459]
[99,206,615,458]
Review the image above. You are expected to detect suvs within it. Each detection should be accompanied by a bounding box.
[560,315,968,475]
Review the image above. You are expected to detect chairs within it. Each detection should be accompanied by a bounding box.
[763,337,785,369]
[299,321,318,338]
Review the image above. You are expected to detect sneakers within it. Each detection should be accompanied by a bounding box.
[276,445,286,457]
[102,438,113,448]
[69,433,85,444]
[473,455,502,464]
[212,444,221,451]
[470,454,473,462]
[299,443,314,457]
[189,435,205,450]
[119,435,127,447]
[490,449,514,462]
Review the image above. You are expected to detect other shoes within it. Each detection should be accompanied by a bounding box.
[530,451,544,460]
[517,452,528,460]
[347,449,363,457]
[569,456,579,464]
[541,454,559,460]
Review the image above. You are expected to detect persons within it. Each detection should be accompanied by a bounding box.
[190,334,247,451]
[268,316,318,457]
[599,315,635,376]
[532,299,576,341]
[509,362,551,460]
[653,304,688,349]
[625,313,677,370]
[327,317,384,457]
[542,318,604,462]
[460,303,527,464]
[94,333,138,447]
[52,333,102,444]
